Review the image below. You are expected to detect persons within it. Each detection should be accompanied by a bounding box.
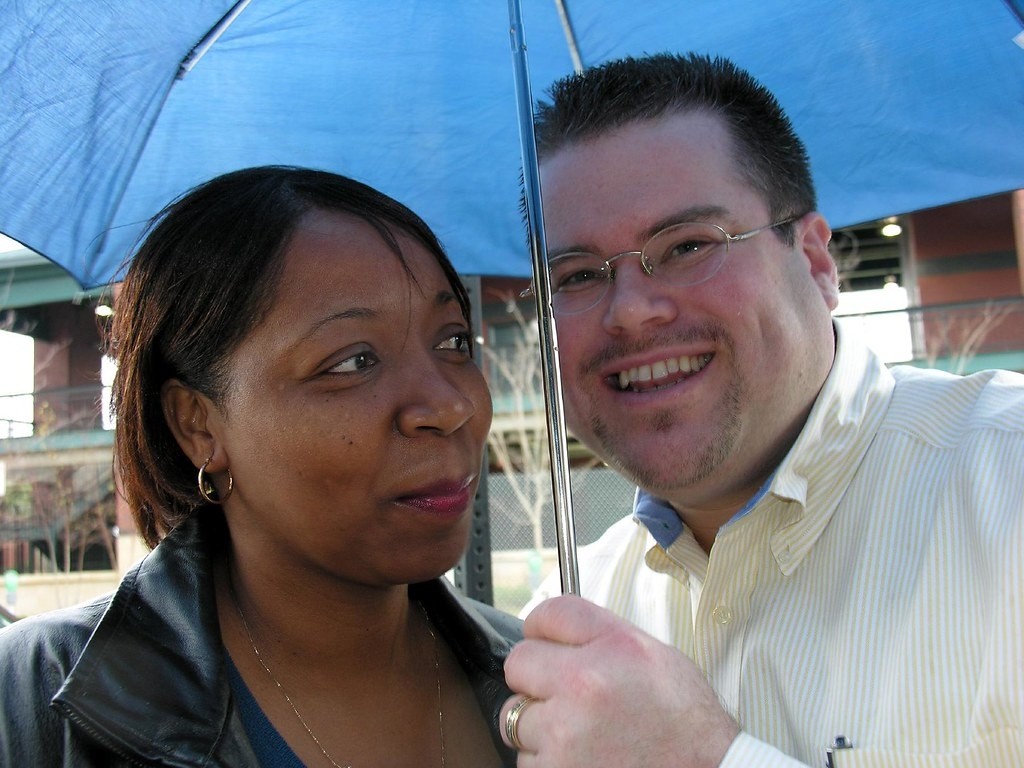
[0,165,526,768]
[482,52,1024,768]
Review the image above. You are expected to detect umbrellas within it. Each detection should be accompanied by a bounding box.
[0,0,1024,597]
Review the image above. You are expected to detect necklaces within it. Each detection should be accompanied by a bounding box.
[229,579,445,768]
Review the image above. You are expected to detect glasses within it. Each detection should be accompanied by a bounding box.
[519,218,796,316]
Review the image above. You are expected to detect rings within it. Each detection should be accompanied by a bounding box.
[506,696,538,750]
